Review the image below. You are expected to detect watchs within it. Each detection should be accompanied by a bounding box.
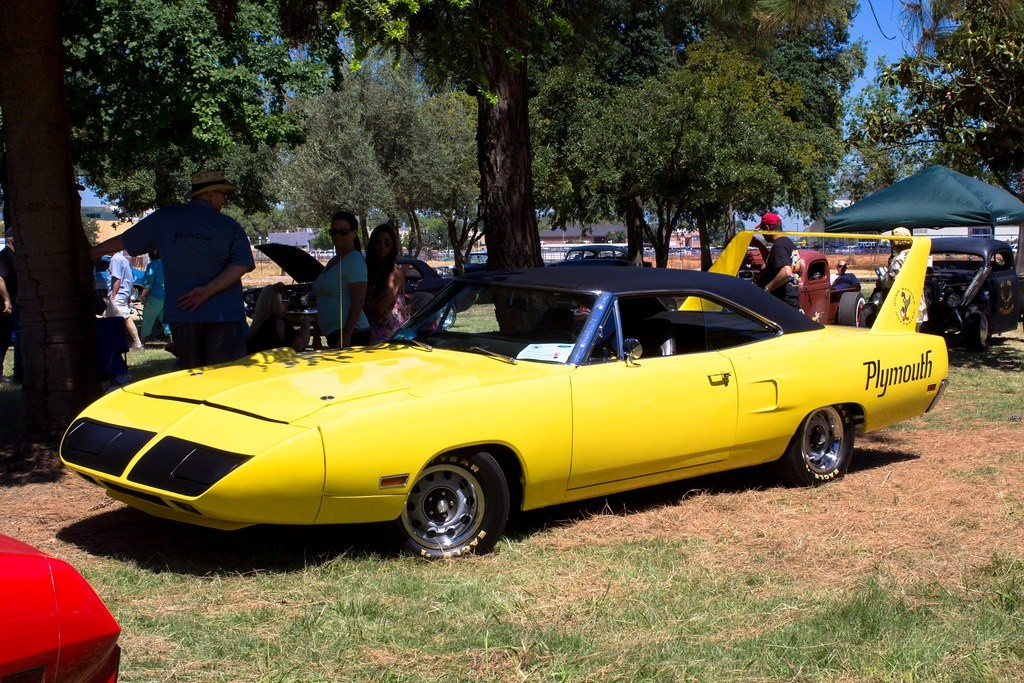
[764,287,768,291]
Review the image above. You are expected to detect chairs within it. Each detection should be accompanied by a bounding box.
[513,309,576,339]
[621,301,674,356]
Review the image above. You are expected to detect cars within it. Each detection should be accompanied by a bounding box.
[859,235,1024,350]
[559,246,653,267]
[245,242,480,330]
[736,247,866,327]
[1,533,121,683]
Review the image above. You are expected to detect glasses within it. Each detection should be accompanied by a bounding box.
[374,238,394,247]
[212,189,229,198]
[282,297,291,304]
[838,265,845,269]
[328,227,355,236]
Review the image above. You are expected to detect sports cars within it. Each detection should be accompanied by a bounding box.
[58,229,950,562]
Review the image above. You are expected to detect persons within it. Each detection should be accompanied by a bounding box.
[246,282,314,355]
[106,253,142,349]
[0,228,17,383]
[313,211,371,347]
[91,170,255,369]
[756,212,803,311]
[363,225,407,346]
[887,227,928,331]
[802,239,806,246]
[832,260,859,289]
[139,250,172,346]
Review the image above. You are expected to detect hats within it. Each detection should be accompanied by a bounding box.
[6,227,13,237]
[186,170,238,198]
[756,212,780,229]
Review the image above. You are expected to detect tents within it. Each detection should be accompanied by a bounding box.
[821,164,1024,255]
